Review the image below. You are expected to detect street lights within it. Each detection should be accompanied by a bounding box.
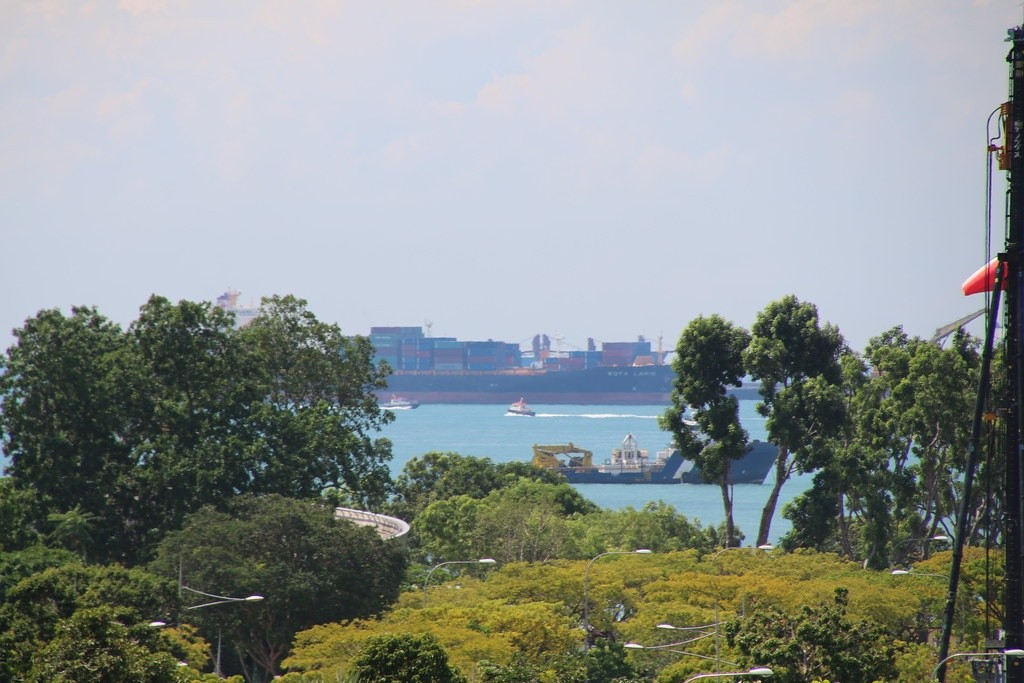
[176,596,265,632]
[929,649,1024,683]
[656,614,745,638]
[888,535,949,570]
[623,631,736,674]
[891,569,965,644]
[684,668,774,683]
[712,544,776,580]
[423,557,498,610]
[584,548,652,651]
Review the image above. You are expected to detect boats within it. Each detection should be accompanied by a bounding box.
[383,392,420,409]
[508,397,536,415]
[528,407,780,486]
